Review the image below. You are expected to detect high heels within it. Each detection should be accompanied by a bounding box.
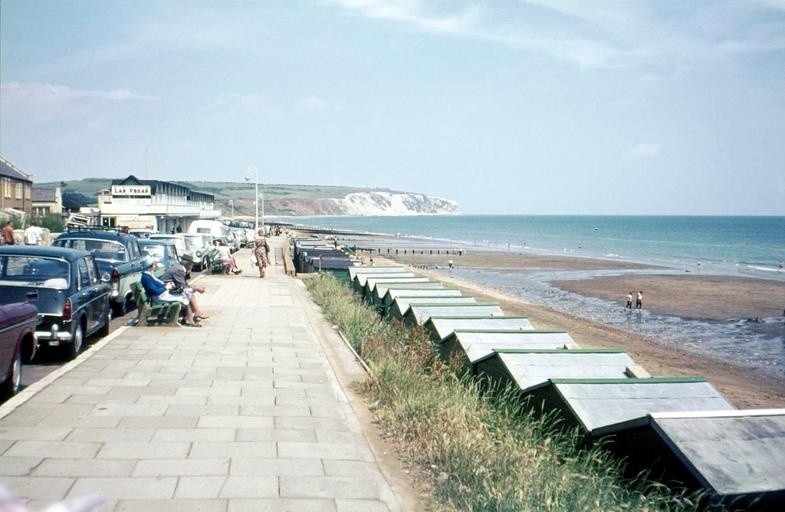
[184,313,209,327]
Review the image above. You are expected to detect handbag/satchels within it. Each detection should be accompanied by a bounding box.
[265,238,270,252]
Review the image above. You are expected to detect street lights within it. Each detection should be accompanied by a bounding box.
[244,165,259,237]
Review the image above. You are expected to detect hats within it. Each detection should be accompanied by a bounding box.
[179,253,196,263]
[139,255,156,269]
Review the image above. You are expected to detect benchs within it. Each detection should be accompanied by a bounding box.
[129,268,182,326]
[200,250,228,275]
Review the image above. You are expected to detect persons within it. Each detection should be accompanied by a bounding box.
[370,256,374,266]
[459,248,463,256]
[140,256,209,328]
[22,223,41,245]
[447,259,454,272]
[625,290,633,310]
[213,224,283,278]
[333,235,337,248]
[32,222,43,245]
[1,220,16,245]
[636,290,643,308]
[158,253,205,322]
